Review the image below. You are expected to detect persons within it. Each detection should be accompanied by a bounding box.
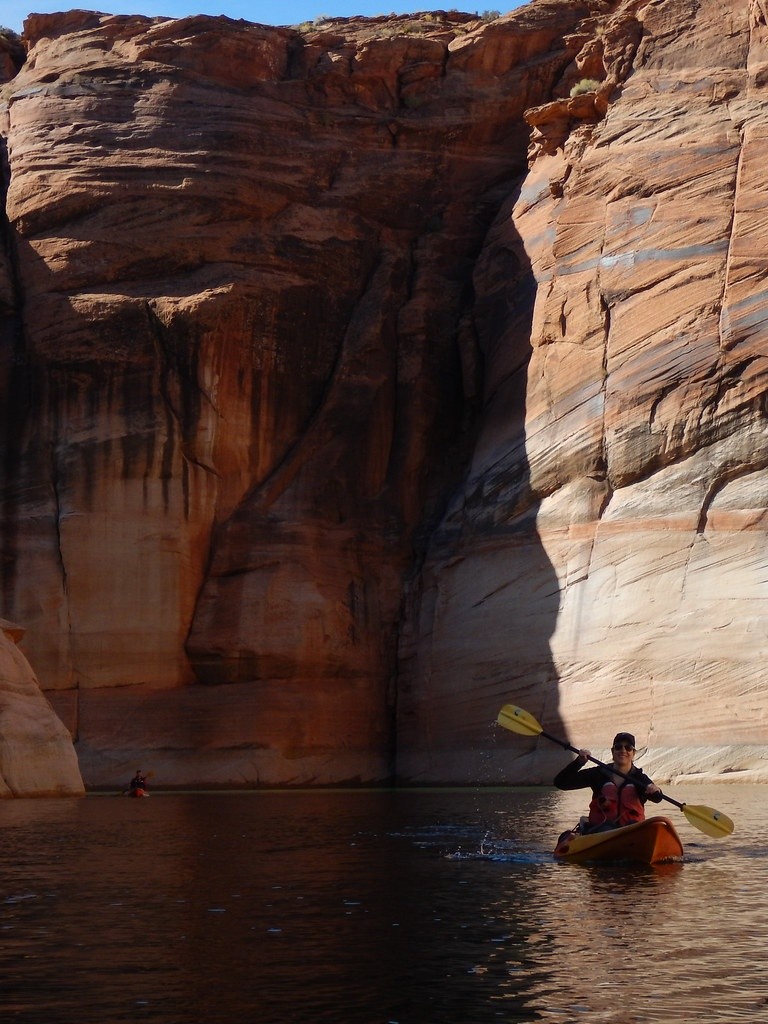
[128,770,148,790]
[553,732,663,835]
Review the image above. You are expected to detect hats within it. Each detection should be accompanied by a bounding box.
[613,732,635,747]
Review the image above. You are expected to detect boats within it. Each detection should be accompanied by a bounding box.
[129,788,150,799]
[553,816,684,866]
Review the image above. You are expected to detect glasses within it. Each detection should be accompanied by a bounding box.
[614,743,635,751]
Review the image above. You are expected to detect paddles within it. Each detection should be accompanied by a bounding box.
[496,703,735,840]
